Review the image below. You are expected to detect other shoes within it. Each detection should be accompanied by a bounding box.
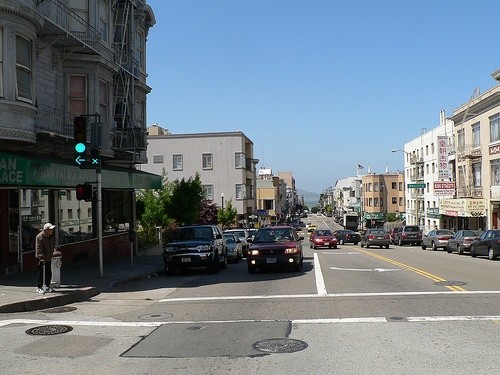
[37,287,44,293]
[44,286,55,292]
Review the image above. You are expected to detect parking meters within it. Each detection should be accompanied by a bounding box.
[128,230,136,271]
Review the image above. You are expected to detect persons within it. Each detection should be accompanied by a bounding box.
[492,225,497,229]
[36,223,56,294]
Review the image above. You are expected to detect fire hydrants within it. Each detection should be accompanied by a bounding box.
[51,249,62,288]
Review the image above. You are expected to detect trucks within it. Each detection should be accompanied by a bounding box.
[343,214,358,230]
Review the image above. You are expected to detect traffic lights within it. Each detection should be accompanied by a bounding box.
[74,116,86,164]
[76,184,93,200]
[90,146,100,167]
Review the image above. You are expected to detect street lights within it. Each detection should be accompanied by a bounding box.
[220,193,224,211]
[392,149,418,229]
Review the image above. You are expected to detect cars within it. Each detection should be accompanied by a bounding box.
[333,230,361,245]
[293,212,307,224]
[223,234,243,263]
[306,222,317,232]
[446,230,480,255]
[317,211,332,217]
[421,229,455,251]
[294,225,301,231]
[388,224,423,246]
[334,216,343,226]
[310,229,337,249]
[469,230,500,260]
[360,229,390,248]
[223,229,258,258]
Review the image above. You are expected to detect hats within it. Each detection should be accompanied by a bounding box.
[44,223,56,230]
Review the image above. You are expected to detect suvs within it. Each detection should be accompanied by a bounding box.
[247,224,305,274]
[162,225,227,274]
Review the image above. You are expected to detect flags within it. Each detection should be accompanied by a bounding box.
[358,164,363,169]
